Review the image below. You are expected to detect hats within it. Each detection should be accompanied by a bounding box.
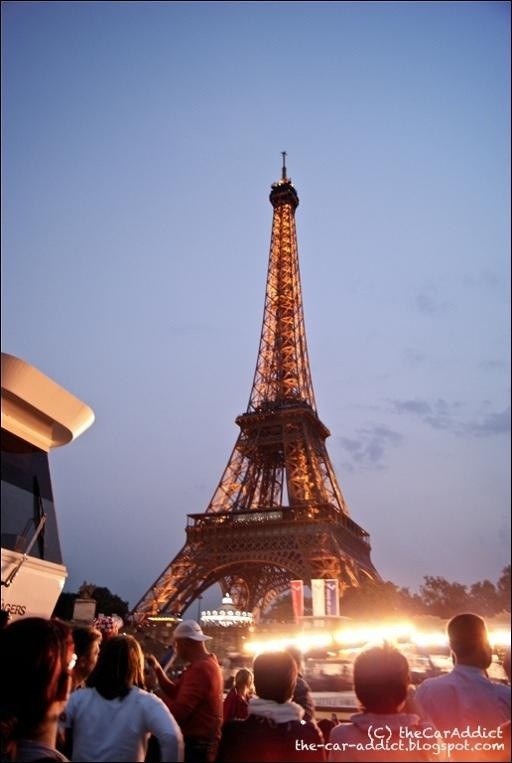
[173,620,213,642]
[95,616,123,634]
